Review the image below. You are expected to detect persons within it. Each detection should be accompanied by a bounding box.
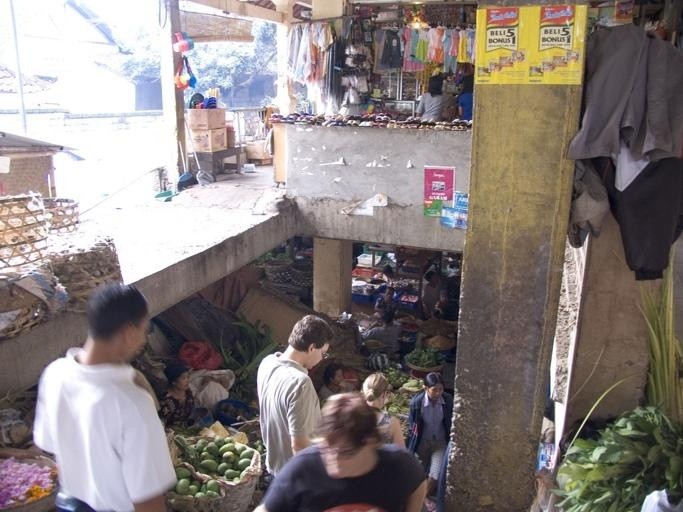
[352,244,461,370]
[406,371,454,512]
[257,313,334,481]
[251,391,429,511]
[318,363,353,408]
[415,69,474,123]
[32,280,177,511]
[539,399,555,446]
[641,488,683,511]
[361,373,405,448]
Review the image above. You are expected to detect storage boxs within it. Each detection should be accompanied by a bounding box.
[245,140,270,160]
[187,107,227,152]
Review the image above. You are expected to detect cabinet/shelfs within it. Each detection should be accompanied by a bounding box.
[234,107,279,166]
[379,70,424,118]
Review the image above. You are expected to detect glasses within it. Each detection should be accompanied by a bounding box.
[322,351,330,360]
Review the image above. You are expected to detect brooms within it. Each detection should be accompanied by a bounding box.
[178,142,198,192]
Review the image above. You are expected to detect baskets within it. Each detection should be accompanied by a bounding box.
[0,189,81,343]
[418,318,456,353]
[43,232,124,318]
[0,439,60,512]
[162,417,274,512]
[263,257,314,289]
[403,352,447,373]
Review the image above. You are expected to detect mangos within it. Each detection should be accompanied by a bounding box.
[175,436,255,500]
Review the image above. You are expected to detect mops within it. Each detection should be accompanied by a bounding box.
[186,121,215,187]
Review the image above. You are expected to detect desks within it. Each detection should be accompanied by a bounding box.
[191,146,241,183]
